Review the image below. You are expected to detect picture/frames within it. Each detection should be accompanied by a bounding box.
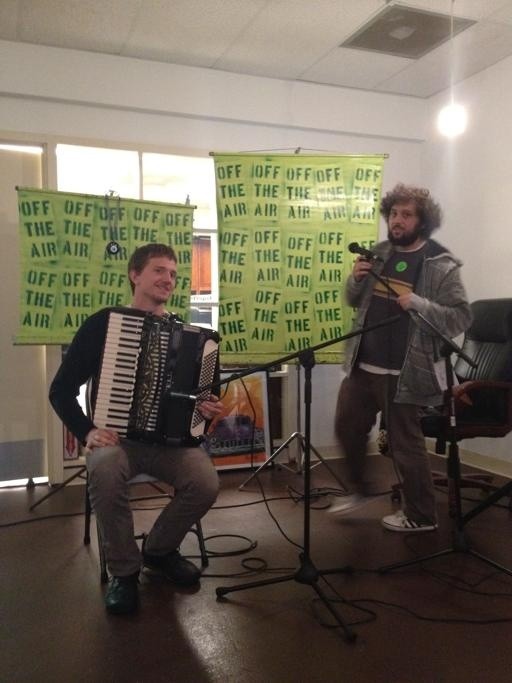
[209,368,274,472]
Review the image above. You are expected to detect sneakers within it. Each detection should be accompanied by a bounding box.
[382,512,438,532]
[105,575,137,614]
[140,533,201,588]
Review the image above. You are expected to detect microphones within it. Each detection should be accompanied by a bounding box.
[165,388,199,403]
[346,241,386,264]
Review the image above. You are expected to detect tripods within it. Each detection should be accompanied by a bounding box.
[216,366,378,644]
[238,380,351,497]
[380,346,510,621]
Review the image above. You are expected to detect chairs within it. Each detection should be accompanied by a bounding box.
[84,378,209,568]
[391,298,512,554]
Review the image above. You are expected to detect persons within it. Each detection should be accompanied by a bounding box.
[326,180,474,534]
[48,242,224,618]
